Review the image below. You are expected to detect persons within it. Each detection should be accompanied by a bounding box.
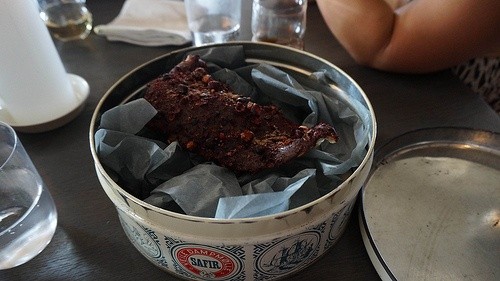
[315,0,500,120]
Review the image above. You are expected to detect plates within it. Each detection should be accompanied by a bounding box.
[357,128,499,281]
[1,73,91,134]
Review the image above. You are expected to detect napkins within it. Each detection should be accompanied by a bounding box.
[93,0,194,46]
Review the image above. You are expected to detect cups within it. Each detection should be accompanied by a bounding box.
[1,0,76,122]
[252,0,307,52]
[38,1,93,42]
[185,0,240,46]
[0,120,57,270]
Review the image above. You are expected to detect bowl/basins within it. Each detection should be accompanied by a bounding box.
[89,41,378,280]
